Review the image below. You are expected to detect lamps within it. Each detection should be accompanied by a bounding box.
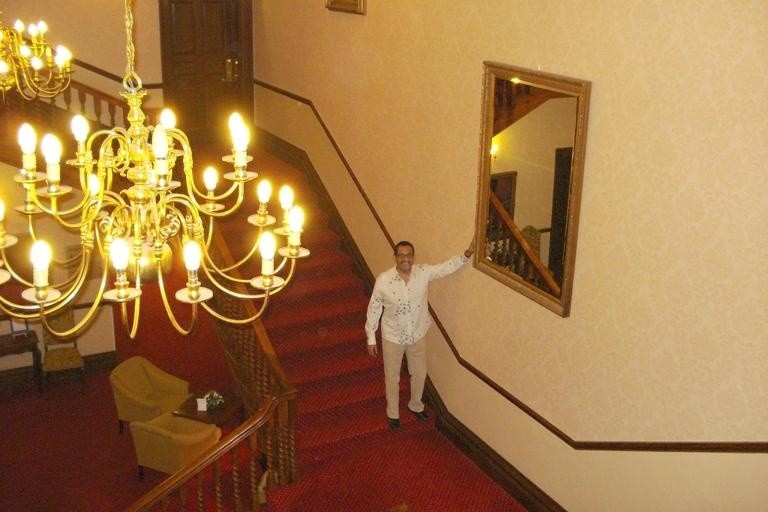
[0,16,74,102]
[0,0,312,339]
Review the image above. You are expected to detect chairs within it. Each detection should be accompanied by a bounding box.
[42,306,85,394]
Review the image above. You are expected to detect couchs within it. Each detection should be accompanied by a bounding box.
[108,356,193,434]
[129,413,222,479]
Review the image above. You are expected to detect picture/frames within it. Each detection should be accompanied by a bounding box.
[487,171,518,240]
[324,0,367,16]
[10,309,29,334]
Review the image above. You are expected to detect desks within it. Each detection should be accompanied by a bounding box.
[0,330,43,393]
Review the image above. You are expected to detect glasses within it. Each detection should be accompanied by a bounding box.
[398,252,415,259]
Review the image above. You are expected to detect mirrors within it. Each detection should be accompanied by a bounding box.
[472,60,593,318]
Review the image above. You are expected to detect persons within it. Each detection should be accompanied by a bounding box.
[364,241,474,432]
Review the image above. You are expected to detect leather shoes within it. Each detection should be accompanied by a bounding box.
[418,410,429,420]
[389,415,401,429]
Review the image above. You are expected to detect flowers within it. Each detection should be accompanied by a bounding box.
[204,392,223,409]
